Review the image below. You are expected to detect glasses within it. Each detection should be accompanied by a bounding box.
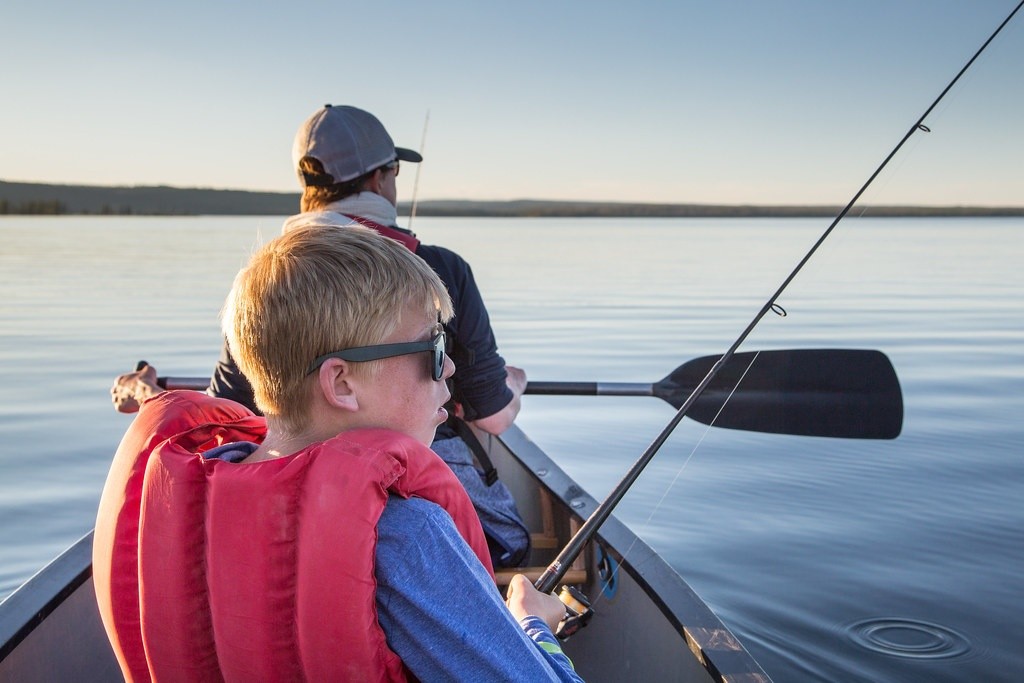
[358,157,400,181]
[305,323,446,381]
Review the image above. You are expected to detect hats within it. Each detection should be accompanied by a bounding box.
[291,102,423,186]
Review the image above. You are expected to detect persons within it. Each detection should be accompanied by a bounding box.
[92,224,585,683]
[112,103,532,569]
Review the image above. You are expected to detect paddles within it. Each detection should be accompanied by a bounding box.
[136,348,905,440]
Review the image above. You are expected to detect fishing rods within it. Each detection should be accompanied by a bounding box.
[533,2,1023,641]
